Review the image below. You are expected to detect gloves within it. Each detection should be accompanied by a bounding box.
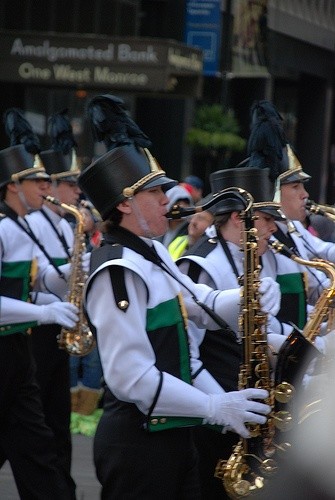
[0,295,79,336]
[145,365,271,438]
[40,248,91,295]
[200,277,281,330]
[266,317,335,375]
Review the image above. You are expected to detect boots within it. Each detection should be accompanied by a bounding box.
[70,382,104,436]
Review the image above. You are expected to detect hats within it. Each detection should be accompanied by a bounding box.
[236,142,313,184]
[77,143,179,223]
[0,143,52,186]
[40,145,81,181]
[209,167,288,223]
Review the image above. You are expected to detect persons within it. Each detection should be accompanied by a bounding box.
[0,104,335,500]
[78,144,274,500]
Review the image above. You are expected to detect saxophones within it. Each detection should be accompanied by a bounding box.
[41,192,101,358]
[167,186,325,500]
[267,199,335,341]
